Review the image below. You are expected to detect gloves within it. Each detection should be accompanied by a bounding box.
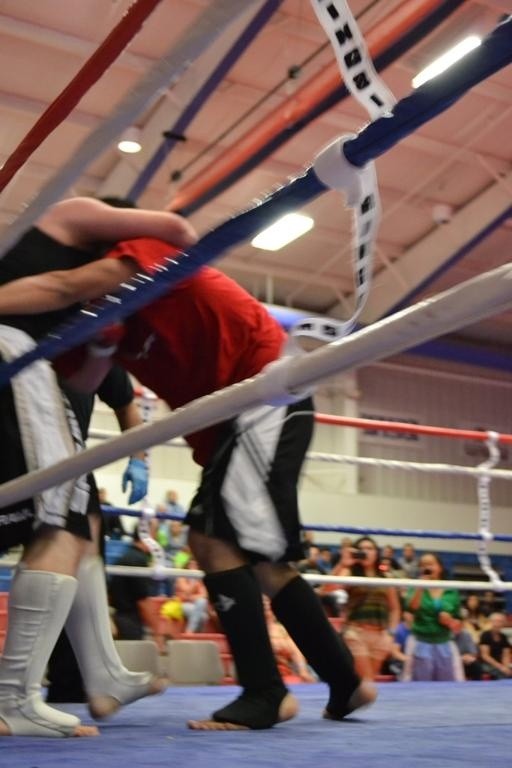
[90,319,123,358]
[123,458,150,505]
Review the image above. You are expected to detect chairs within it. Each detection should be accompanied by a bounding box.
[112,639,224,685]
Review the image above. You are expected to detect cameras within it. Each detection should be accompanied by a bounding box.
[353,550,367,561]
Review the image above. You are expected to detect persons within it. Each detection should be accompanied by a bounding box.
[261,529,512,684]
[97,488,220,642]
[0,194,200,738]
[1,235,380,729]
